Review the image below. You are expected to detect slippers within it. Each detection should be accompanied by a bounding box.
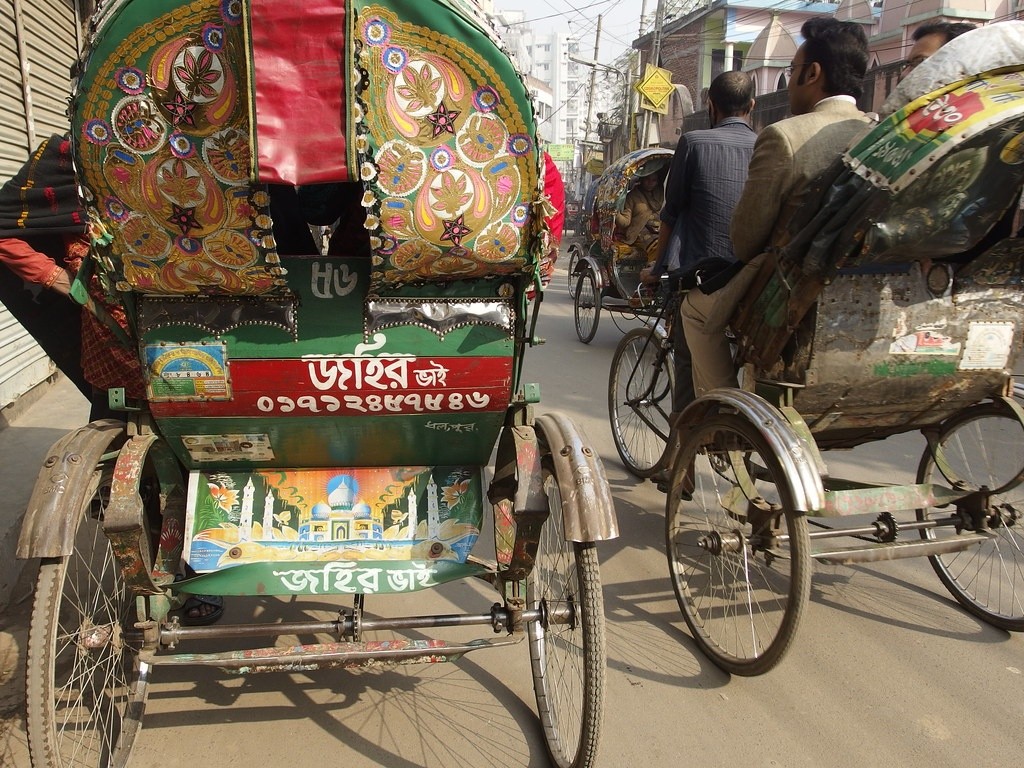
[651,469,692,501]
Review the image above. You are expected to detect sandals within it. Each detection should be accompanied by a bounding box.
[183,594,224,625]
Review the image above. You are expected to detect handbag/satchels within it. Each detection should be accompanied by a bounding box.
[668,256,733,291]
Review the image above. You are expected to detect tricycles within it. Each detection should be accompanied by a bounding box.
[13,0,621,768]
[607,18,1024,677]
[566,147,679,345]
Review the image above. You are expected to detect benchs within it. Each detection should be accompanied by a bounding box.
[588,200,649,264]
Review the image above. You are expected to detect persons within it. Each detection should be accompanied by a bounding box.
[639,71,760,496]
[680,18,872,402]
[0,131,225,626]
[897,19,979,85]
[615,170,665,298]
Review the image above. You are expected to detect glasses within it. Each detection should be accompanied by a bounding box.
[902,57,927,71]
[784,62,823,76]
